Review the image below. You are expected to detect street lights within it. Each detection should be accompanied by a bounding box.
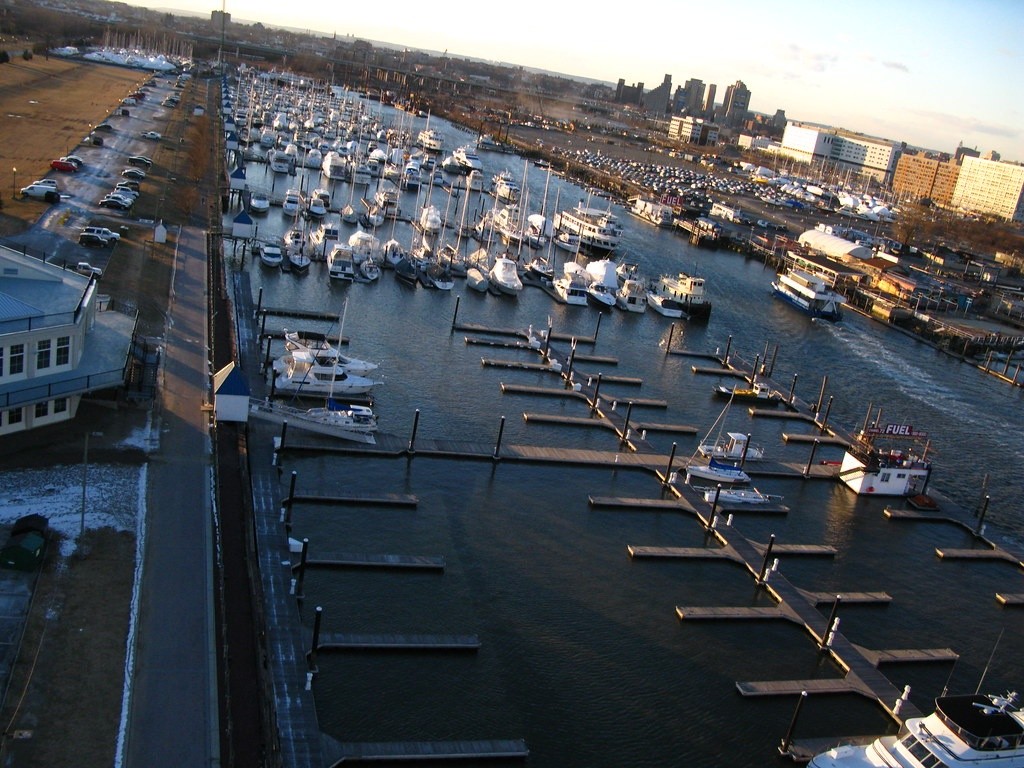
[79,430,104,558]
[12,166,17,199]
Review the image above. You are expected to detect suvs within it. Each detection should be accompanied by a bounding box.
[32,179,58,187]
[79,233,109,249]
[50,22,713,431]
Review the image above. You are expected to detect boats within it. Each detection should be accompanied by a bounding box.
[698,432,765,463]
[703,432,792,514]
[806,624,1023,768]
[770,265,849,322]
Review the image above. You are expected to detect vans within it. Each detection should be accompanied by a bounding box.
[82,226,120,243]
[19,184,57,200]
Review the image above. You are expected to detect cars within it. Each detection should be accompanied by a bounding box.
[116,180,140,191]
[140,131,162,142]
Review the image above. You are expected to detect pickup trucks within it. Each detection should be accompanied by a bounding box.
[61,262,103,279]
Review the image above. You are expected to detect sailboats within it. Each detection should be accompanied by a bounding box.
[684,383,752,485]
[713,144,908,223]
[711,340,783,406]
